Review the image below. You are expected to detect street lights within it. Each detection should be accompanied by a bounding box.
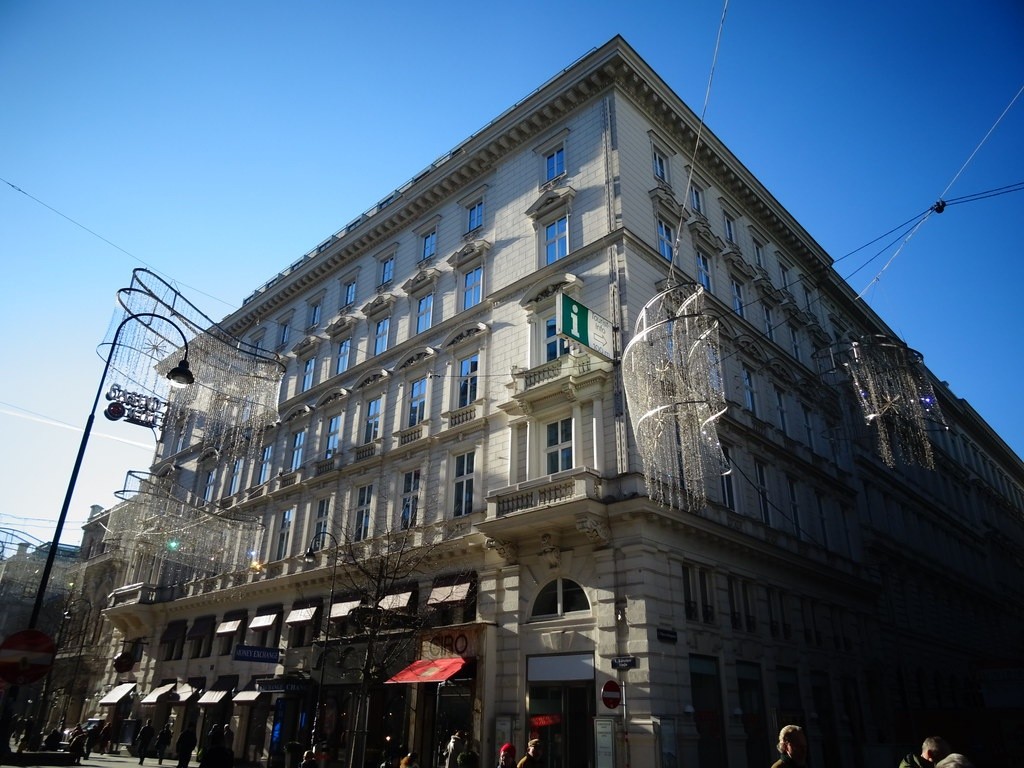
[303,531,339,751]
[1,312,194,755]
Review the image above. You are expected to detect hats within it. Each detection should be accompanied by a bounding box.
[528,739,543,746]
[500,743,516,760]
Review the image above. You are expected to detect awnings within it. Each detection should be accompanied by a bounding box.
[380,656,466,685]
[141,678,177,705]
[249,614,277,629]
[232,690,260,701]
[427,582,470,605]
[327,599,361,619]
[284,606,317,623]
[376,592,412,611]
[168,680,199,704]
[215,620,241,633]
[194,681,231,703]
[99,680,137,705]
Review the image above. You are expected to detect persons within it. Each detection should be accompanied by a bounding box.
[898,736,972,768]
[496,741,517,768]
[134,719,234,768]
[444,731,463,768]
[298,750,318,767]
[380,750,419,768]
[516,739,545,768]
[10,713,121,764]
[769,725,808,768]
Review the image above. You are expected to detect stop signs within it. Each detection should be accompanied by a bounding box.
[0,630,56,686]
[600,679,621,709]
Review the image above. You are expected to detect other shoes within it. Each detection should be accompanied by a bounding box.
[75,761,80,765]
[139,760,143,765]
